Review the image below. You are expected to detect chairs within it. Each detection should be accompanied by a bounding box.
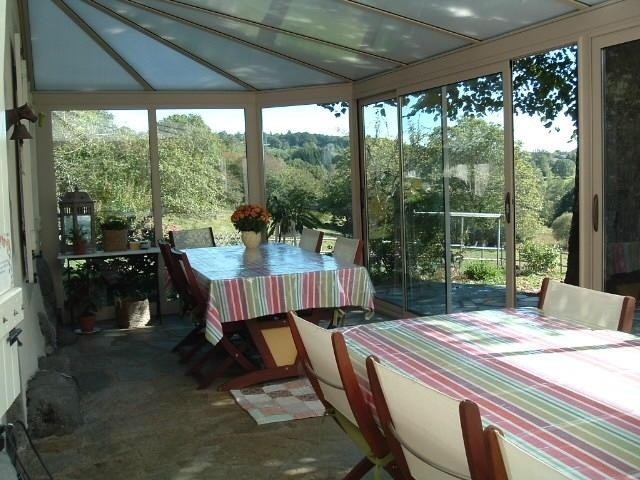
[157,227,368,394]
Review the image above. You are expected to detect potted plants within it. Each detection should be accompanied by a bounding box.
[50,213,163,339]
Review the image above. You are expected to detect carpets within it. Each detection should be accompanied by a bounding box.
[226,375,329,428]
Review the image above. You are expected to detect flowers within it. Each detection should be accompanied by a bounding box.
[229,204,270,225]
